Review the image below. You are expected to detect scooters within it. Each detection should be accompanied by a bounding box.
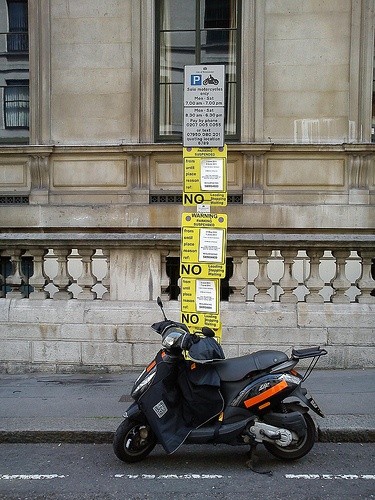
[112,295,328,463]
